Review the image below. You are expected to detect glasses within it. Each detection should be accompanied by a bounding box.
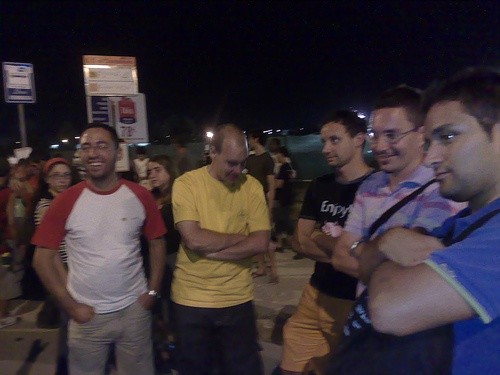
[365,126,423,145]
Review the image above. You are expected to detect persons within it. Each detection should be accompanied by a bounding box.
[268,145,297,249]
[357,66,500,374]
[279,107,382,375]
[168,125,271,375]
[275,145,294,253]
[140,151,182,375]
[243,124,280,285]
[33,158,82,375]
[0,139,219,330]
[29,121,168,375]
[331,97,466,375]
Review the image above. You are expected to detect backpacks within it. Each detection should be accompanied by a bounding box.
[323,203,500,375]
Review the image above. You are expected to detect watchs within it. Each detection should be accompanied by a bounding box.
[148,290,161,299]
[350,239,364,253]
